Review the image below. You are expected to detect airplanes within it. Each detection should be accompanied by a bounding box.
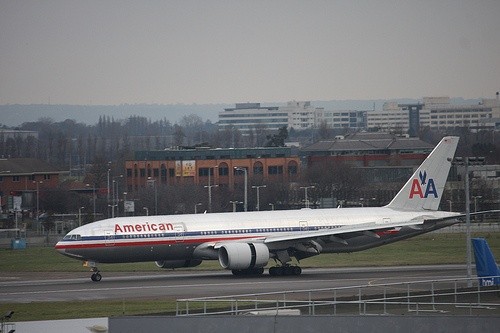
[56,135,464,282]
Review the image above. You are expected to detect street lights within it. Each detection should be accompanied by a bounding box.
[111,175,123,218]
[229,200,243,212]
[147,176,157,215]
[208,165,218,213]
[300,186,315,208]
[269,203,274,210]
[79,207,84,227]
[252,185,267,211]
[86,184,96,222]
[107,169,111,218]
[203,184,219,212]
[472,195,482,220]
[447,200,452,212]
[234,166,247,212]
[32,180,44,234]
[359,198,376,206]
[143,207,148,216]
[194,202,202,214]
